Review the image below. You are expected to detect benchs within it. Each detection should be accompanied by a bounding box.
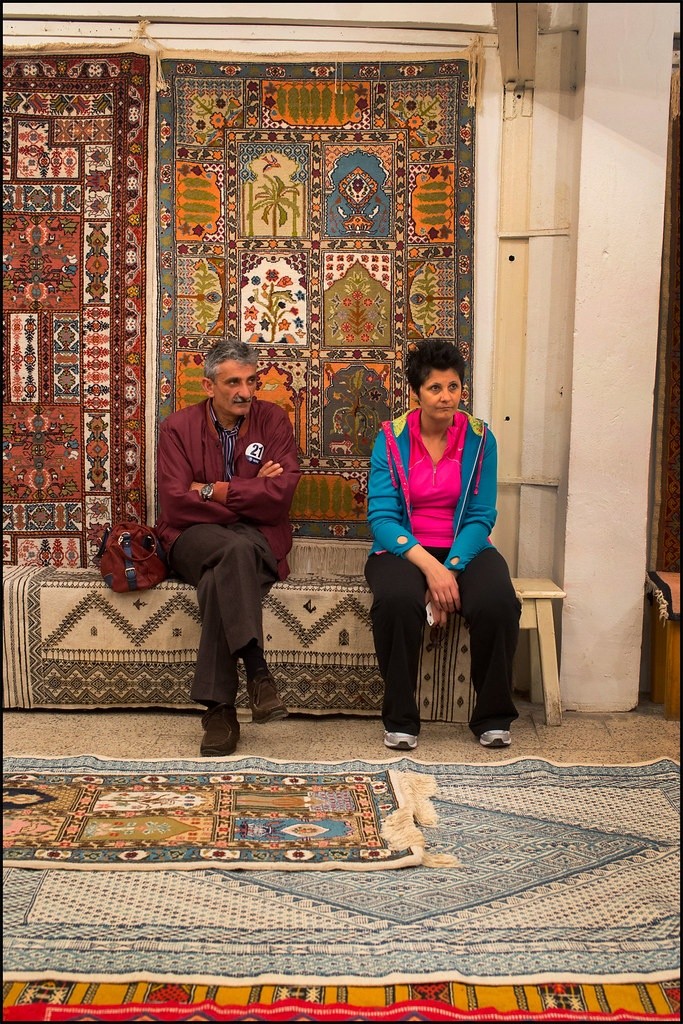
[3,563,564,727]
[648,571,680,721]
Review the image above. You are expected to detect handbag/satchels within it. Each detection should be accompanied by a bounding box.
[92,521,167,593]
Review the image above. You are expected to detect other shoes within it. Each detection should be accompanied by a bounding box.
[246,667,288,724]
[200,706,240,756]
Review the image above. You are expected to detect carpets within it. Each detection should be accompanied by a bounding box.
[3,980,681,1021]
[3,769,460,870]
[3,753,679,985]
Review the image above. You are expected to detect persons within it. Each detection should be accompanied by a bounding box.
[363,338,521,751]
[155,339,302,757]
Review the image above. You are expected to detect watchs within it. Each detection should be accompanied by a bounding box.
[201,483,215,501]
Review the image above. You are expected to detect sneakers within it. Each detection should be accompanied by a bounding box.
[383,728,418,749]
[479,730,511,746]
